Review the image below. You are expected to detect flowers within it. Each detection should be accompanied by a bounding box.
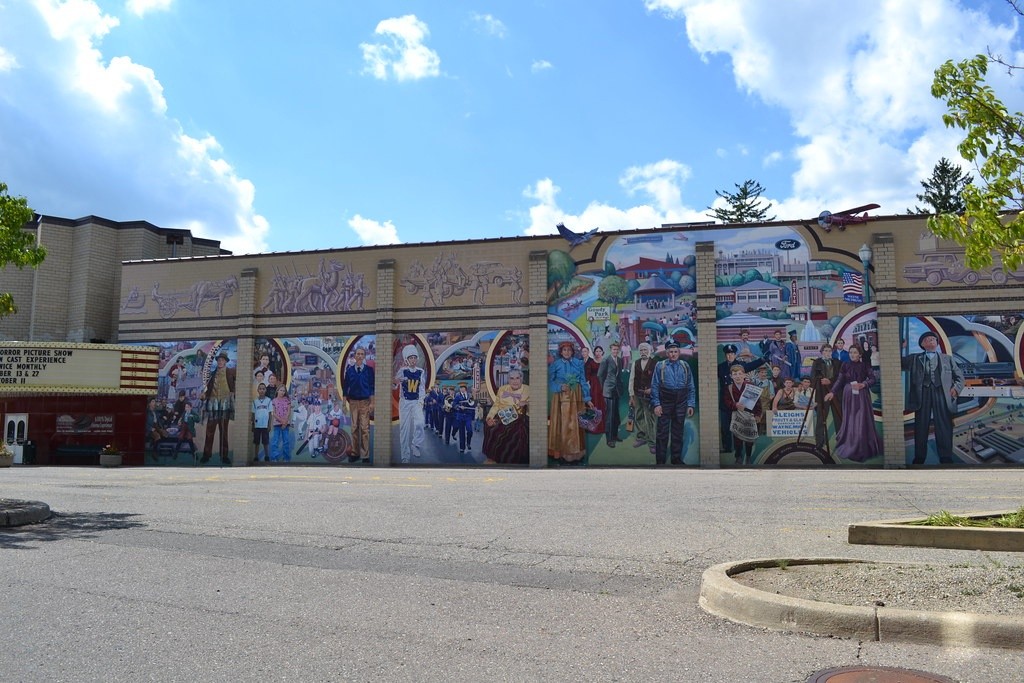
[99,445,125,456]
[0,439,14,455]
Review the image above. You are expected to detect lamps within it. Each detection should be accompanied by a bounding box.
[857,240,873,304]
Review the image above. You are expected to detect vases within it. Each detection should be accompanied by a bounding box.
[0,456,14,466]
[100,455,122,467]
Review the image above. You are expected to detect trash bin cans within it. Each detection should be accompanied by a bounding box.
[23,440,36,465]
